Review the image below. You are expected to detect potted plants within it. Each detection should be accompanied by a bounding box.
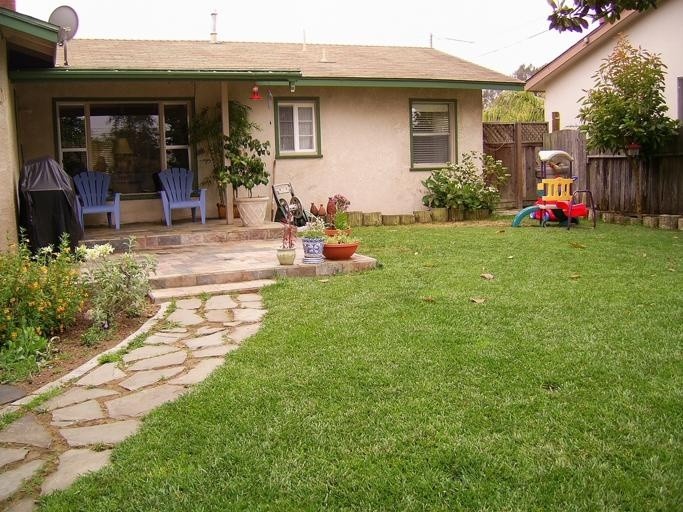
[186,100,272,228]
[419,150,512,221]
[276,193,359,265]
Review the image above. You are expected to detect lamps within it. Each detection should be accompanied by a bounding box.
[248,80,265,101]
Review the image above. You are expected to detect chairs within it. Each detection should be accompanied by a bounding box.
[156,167,208,228]
[74,170,120,229]
